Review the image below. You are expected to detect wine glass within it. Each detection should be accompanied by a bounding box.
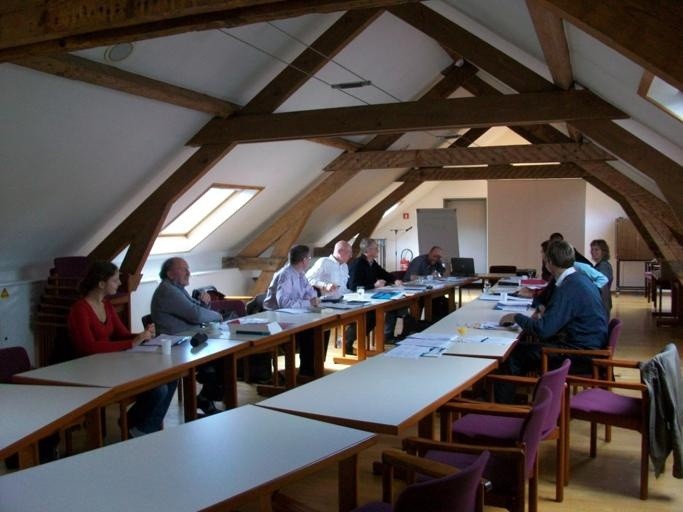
[527,268,532,279]
[456,321,467,344]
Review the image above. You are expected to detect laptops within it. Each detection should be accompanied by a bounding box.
[450,258,478,277]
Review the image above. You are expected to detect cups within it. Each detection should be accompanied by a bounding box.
[159,338,171,355]
[500,291,507,301]
[417,275,423,284]
[426,275,433,283]
[356,286,364,295]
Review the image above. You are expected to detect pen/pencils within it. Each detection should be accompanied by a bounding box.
[481,337,489,342]
[141,326,151,346]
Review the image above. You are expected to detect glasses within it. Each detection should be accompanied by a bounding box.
[303,256,311,260]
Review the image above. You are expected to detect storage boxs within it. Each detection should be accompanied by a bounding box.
[615,217,656,261]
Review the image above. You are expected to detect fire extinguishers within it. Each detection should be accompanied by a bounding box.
[400,249,413,272]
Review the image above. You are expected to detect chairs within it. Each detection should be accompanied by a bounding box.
[524,314,618,448]
[340,448,488,510]
[400,388,551,512]
[446,360,571,512]
[563,344,676,499]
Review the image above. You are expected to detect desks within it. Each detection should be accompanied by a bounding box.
[1,276,536,345]
[0,382,113,476]
[15,334,251,448]
[616,258,683,328]
[256,351,499,510]
[1,402,380,512]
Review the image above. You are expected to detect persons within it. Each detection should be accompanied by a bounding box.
[151,258,225,416]
[346,238,416,345]
[304,240,376,356]
[65,260,179,442]
[259,245,330,377]
[402,246,450,323]
[494,233,613,404]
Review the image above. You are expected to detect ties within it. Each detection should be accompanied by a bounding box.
[370,261,372,266]
[298,273,304,293]
